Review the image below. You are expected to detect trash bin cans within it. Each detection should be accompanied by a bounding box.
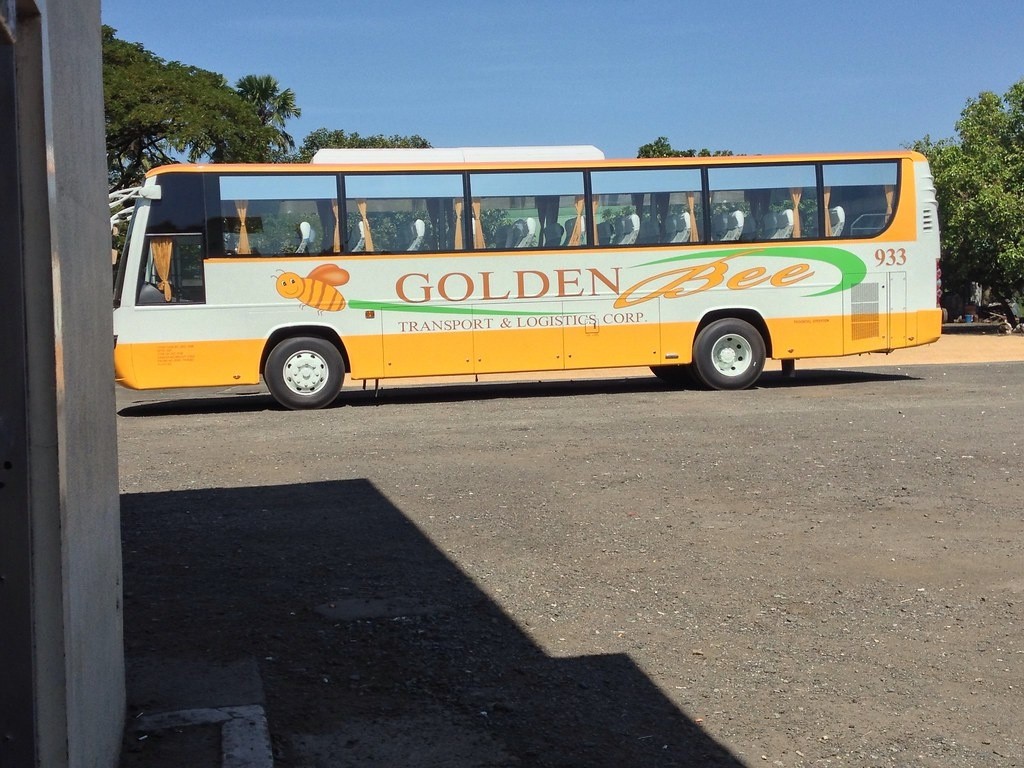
[942,292,963,319]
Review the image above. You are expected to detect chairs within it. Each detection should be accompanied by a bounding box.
[597,213,660,246]
[494,217,536,247]
[543,222,564,246]
[295,220,311,253]
[760,209,794,239]
[808,206,846,237]
[665,211,692,244]
[401,218,426,250]
[710,209,757,241]
[346,220,368,252]
[563,215,588,248]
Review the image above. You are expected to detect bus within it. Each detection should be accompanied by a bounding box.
[107,146,946,406]
